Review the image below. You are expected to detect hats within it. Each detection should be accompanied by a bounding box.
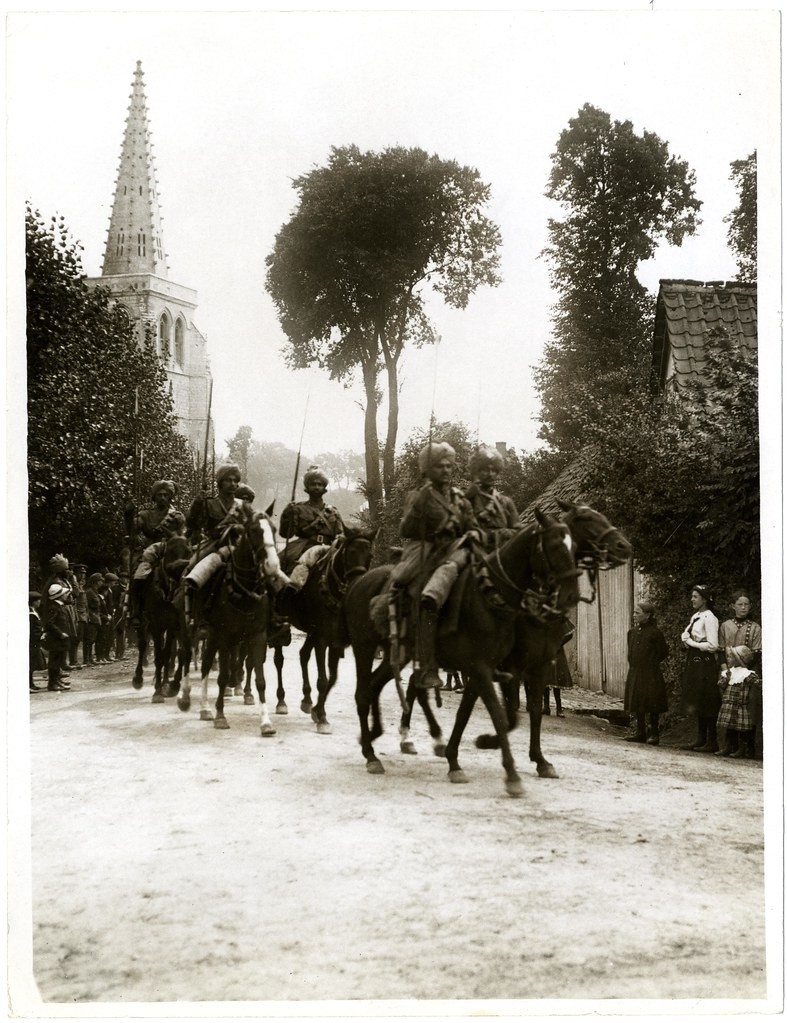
[151,480,181,500]
[468,447,505,474]
[304,466,329,488]
[49,554,69,572]
[730,645,753,668]
[29,591,43,601]
[72,564,88,574]
[418,442,456,476]
[89,573,104,581]
[105,573,119,581]
[693,584,714,603]
[118,572,130,577]
[217,463,242,485]
[233,481,255,501]
[48,584,70,600]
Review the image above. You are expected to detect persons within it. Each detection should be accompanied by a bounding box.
[719,592,762,651]
[186,464,267,547]
[400,442,471,688]
[717,645,762,756]
[465,447,521,546]
[280,469,345,553]
[624,604,669,743]
[681,586,718,753]
[236,483,255,504]
[30,553,132,692]
[126,480,185,628]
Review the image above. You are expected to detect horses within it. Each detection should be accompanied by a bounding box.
[132,524,290,707]
[169,496,282,738]
[396,496,634,759]
[271,518,383,735]
[341,504,584,796]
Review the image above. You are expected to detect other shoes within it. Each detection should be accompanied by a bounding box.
[29,656,129,694]
[714,743,732,755]
[626,734,659,746]
[729,745,746,758]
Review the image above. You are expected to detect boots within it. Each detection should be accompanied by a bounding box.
[680,725,705,750]
[694,726,718,753]
[414,607,444,689]
